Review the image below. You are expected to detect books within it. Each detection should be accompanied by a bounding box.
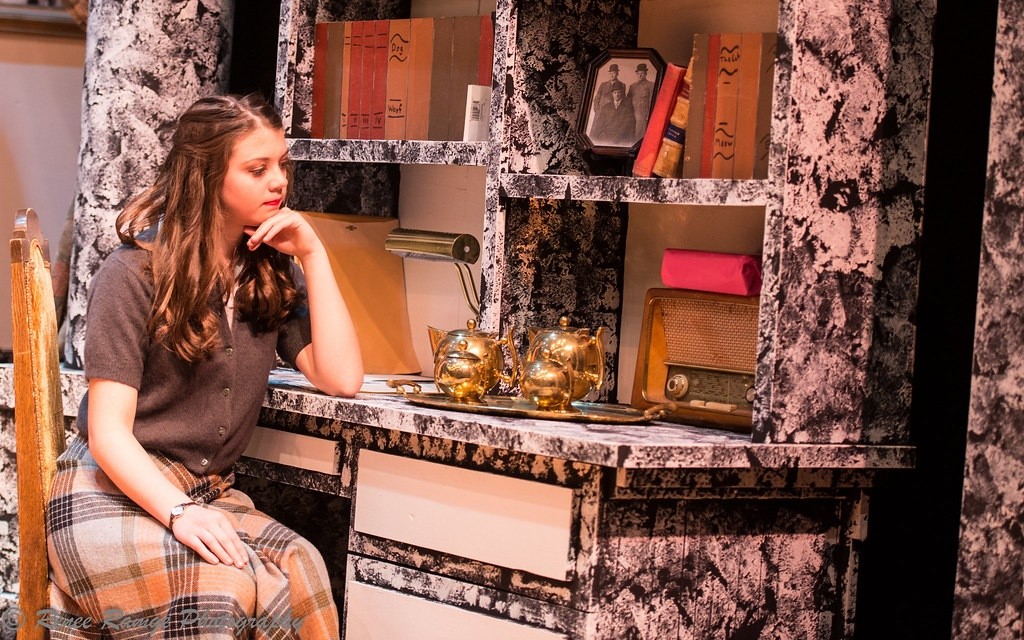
[309,11,493,141]
[632,32,776,181]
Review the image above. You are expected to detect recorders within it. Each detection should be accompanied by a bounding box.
[631,287,758,430]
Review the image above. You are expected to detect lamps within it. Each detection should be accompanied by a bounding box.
[385,227,480,318]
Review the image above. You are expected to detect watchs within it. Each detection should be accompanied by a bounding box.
[168,502,198,530]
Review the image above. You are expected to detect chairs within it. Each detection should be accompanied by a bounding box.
[9,206,67,640]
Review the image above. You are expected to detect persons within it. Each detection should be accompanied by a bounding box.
[591,64,625,111]
[47,92,362,640]
[590,82,636,145]
[626,62,654,138]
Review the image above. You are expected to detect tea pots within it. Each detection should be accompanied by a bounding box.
[520,349,574,413]
[524,315,606,407]
[425,316,518,396]
[432,339,488,404]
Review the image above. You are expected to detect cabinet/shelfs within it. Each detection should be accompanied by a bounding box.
[229,0,938,640]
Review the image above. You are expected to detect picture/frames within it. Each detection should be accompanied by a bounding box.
[575,47,668,156]
